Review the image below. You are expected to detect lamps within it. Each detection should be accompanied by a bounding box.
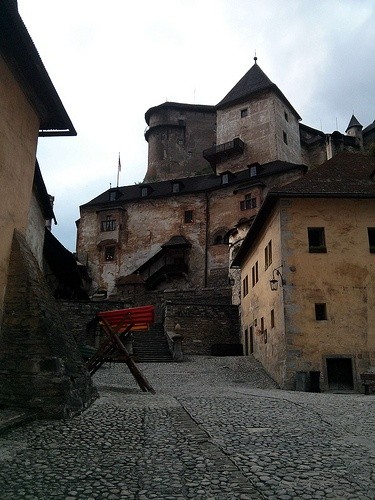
[268,264,287,291]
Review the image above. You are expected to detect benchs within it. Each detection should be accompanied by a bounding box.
[360,373,375,396]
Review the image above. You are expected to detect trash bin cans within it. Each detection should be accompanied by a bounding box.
[296,370,321,393]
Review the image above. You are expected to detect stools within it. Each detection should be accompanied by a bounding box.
[84,304,161,396]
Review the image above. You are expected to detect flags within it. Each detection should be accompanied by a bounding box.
[119,154,122,172]
